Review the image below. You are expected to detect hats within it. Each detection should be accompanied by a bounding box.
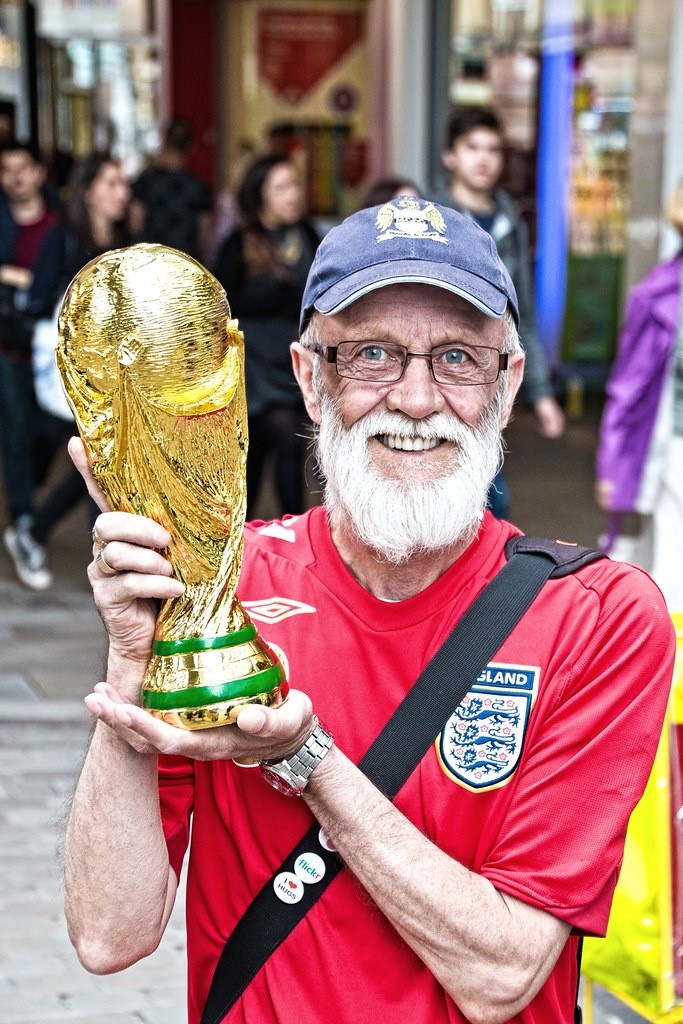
[298,195,520,343]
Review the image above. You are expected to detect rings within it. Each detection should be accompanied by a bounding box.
[92,527,107,548]
[96,550,116,575]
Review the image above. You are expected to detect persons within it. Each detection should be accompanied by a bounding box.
[63,197,676,1024]
[0,109,683,608]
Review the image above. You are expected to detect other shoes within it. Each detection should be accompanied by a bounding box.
[3,514,52,590]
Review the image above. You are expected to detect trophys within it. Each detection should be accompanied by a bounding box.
[54,242,291,731]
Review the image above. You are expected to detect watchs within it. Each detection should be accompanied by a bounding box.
[260,715,334,797]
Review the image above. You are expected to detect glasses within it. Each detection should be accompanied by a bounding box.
[302,339,509,386]
